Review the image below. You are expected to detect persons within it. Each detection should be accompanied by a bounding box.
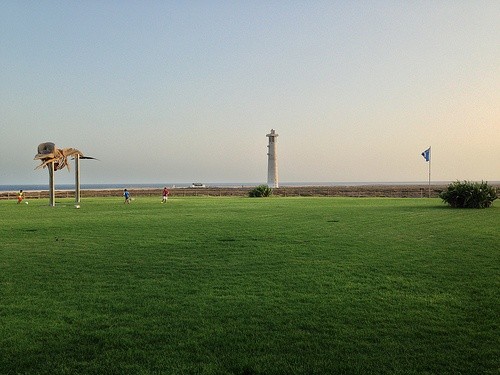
[16,189,24,206]
[160,186,169,205]
[123,188,132,205]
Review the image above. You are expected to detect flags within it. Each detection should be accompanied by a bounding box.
[421,148,430,162]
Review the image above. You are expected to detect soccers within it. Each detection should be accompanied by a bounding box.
[25,202,28,204]
